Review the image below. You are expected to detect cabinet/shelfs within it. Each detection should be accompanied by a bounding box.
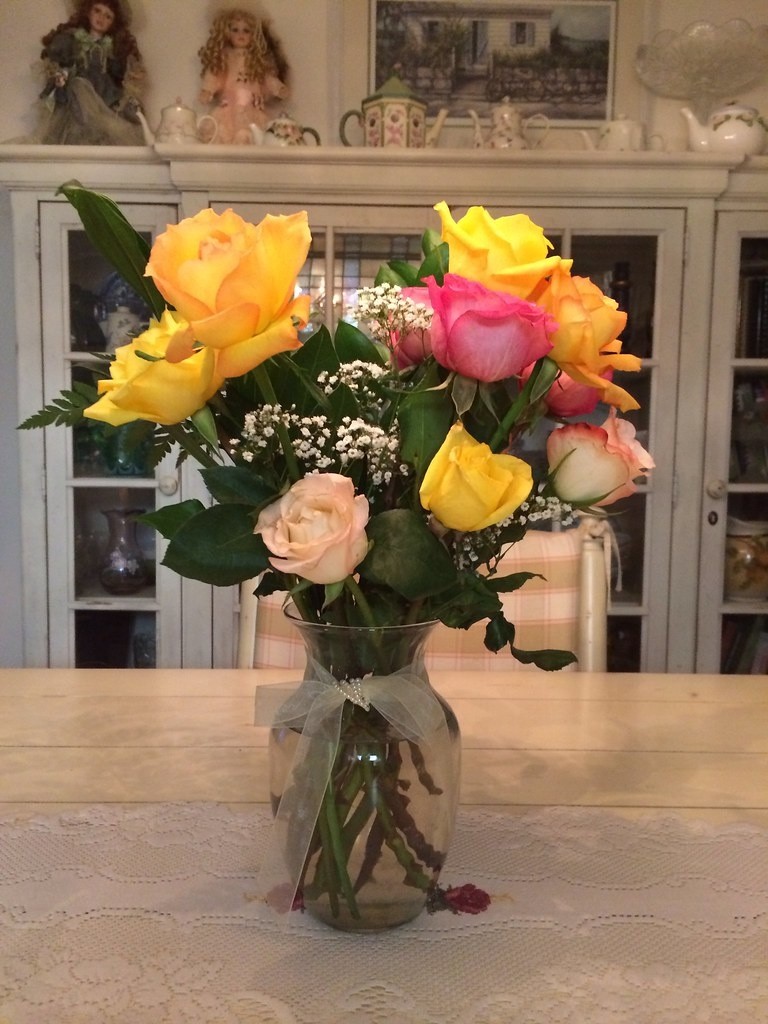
[2,143,768,672]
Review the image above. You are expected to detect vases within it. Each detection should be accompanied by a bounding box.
[270,596,465,937]
[101,508,149,594]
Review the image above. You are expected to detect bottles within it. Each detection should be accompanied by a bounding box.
[609,262,633,353]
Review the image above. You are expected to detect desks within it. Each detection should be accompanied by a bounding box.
[0,669,768,1024]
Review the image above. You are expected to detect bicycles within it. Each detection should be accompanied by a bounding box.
[485,57,608,103]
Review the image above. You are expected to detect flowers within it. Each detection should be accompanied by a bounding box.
[13,175,657,918]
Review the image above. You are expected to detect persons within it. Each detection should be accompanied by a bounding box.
[198,9,290,144]
[31,0,153,146]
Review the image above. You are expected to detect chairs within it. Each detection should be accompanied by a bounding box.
[238,515,614,675]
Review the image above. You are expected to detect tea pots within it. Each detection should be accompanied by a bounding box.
[468,95,549,152]
[679,100,768,156]
[578,113,665,153]
[136,98,217,145]
[339,75,449,149]
[248,112,320,147]
[98,306,150,354]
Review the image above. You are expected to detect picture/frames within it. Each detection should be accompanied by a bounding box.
[368,0,619,129]
